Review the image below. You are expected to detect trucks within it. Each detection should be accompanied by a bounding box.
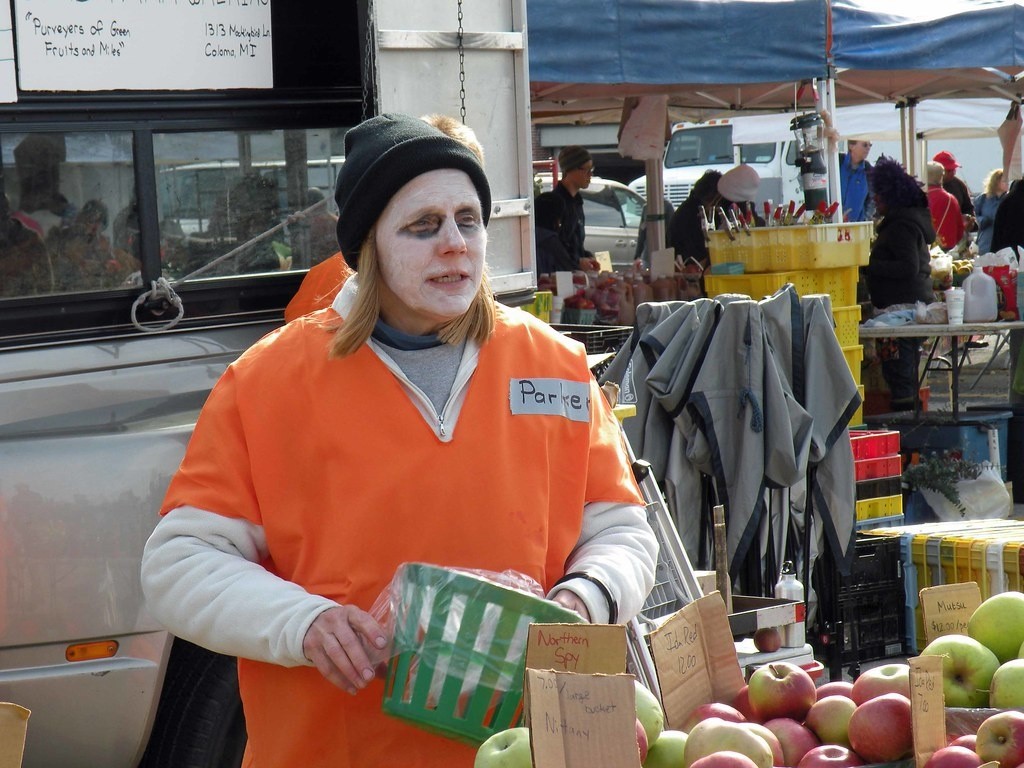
[623,117,846,226]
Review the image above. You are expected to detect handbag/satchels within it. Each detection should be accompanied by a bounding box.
[931,236,943,250]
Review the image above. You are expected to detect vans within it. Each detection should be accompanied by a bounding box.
[533,173,648,279]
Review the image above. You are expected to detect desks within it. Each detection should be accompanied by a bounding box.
[858,320,1024,423]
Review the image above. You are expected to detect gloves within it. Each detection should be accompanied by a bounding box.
[981,217,993,229]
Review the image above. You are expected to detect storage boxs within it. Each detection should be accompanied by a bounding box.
[565,308,597,324]
[705,221,1024,668]
[548,322,634,382]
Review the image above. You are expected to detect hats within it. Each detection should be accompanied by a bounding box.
[333,112,491,271]
[718,165,760,202]
[559,146,592,179]
[933,151,962,170]
[927,161,944,175]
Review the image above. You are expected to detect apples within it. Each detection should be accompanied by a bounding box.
[474,590,1024,768]
[952,260,973,274]
[539,276,621,316]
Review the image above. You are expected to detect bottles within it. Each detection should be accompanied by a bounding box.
[775,560,805,648]
[962,266,997,322]
[539,271,557,296]
[573,270,677,325]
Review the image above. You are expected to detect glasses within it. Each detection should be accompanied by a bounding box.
[854,143,872,148]
[579,166,596,173]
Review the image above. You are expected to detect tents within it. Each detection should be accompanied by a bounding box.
[529,0,1024,278]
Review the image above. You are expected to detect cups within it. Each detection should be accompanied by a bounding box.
[551,296,562,323]
[944,291,965,324]
[930,255,955,301]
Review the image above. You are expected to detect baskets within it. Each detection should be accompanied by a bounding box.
[380,563,592,745]
[703,221,905,668]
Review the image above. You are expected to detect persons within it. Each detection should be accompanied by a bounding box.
[864,158,935,411]
[924,161,965,254]
[990,174,1024,504]
[536,146,600,272]
[2,167,340,298]
[140,112,660,768]
[698,163,770,296]
[284,112,486,324]
[973,169,1008,256]
[665,170,724,268]
[838,138,877,223]
[933,151,990,349]
[633,196,674,268]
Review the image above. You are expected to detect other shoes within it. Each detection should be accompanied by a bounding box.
[889,400,923,412]
